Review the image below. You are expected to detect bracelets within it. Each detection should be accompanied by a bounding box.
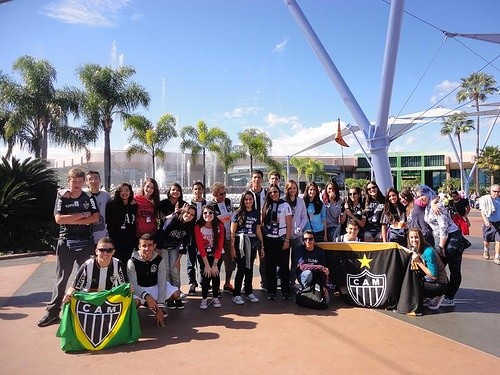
[213,262,217,266]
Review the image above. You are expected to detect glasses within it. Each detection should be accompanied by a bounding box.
[366,186,377,190]
[216,192,227,196]
[349,192,358,196]
[97,248,115,253]
[303,238,315,241]
[269,190,279,194]
[202,211,214,214]
[492,190,500,193]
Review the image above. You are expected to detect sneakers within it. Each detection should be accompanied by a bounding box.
[247,294,259,302]
[208,298,221,307]
[232,296,245,304]
[200,299,208,309]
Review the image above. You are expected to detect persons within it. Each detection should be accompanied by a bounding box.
[63,238,135,302]
[60,170,471,327]
[478,184,500,265]
[37,168,99,327]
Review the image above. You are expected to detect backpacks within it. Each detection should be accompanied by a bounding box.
[296,283,329,307]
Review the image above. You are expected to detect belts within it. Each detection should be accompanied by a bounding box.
[59,233,90,236]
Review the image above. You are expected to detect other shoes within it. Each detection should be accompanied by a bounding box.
[147,310,168,317]
[175,299,184,309]
[189,290,196,295]
[224,285,234,294]
[167,301,176,309]
[494,258,500,265]
[241,288,255,294]
[37,312,59,326]
[281,295,296,302]
[423,294,455,309]
[484,248,490,259]
[264,294,278,300]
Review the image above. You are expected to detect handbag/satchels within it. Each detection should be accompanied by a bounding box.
[67,240,89,250]
[461,216,470,236]
